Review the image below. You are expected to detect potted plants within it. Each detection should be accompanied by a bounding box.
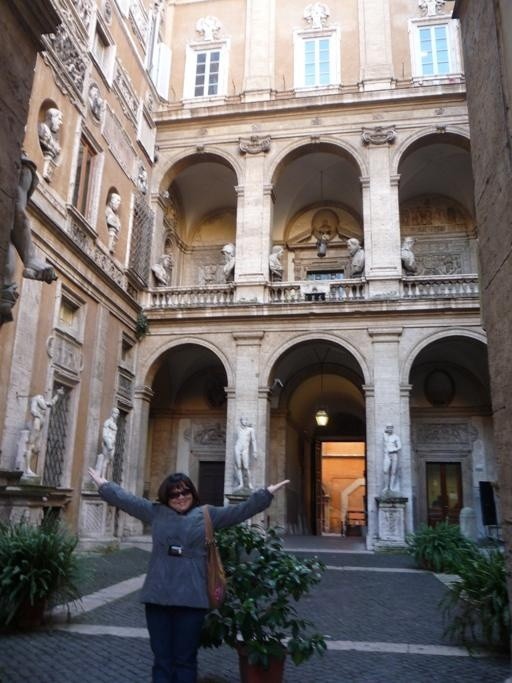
[204,519,335,682]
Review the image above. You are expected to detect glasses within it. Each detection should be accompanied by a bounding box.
[169,488,192,498]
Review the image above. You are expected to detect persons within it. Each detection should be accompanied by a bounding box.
[95,407,122,480]
[153,255,175,285]
[4,153,58,324]
[234,417,259,490]
[87,467,291,683]
[220,243,236,284]
[39,107,64,184]
[382,422,401,492]
[346,237,363,282]
[104,191,121,255]
[401,233,416,285]
[270,242,284,282]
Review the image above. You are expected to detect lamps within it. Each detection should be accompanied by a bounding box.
[317,170,328,257]
[312,347,331,428]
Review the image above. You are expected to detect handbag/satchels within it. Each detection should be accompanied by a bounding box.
[203,504,225,608]
